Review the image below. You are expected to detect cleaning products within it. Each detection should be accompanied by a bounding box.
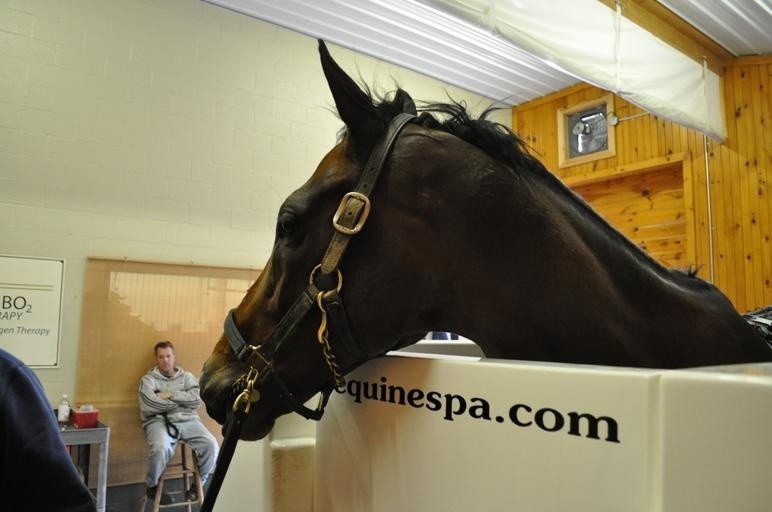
[58,393,70,425]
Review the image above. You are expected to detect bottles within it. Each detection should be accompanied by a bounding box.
[57,394,71,426]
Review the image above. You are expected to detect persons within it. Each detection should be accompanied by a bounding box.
[0,347,98,512]
[138,340,221,505]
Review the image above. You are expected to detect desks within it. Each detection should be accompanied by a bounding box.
[51,407,112,512]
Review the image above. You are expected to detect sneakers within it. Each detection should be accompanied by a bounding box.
[147,486,171,504]
[186,490,197,501]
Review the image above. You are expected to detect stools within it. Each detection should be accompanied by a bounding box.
[137,438,205,512]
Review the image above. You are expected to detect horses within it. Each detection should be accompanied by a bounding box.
[198,39,772,441]
